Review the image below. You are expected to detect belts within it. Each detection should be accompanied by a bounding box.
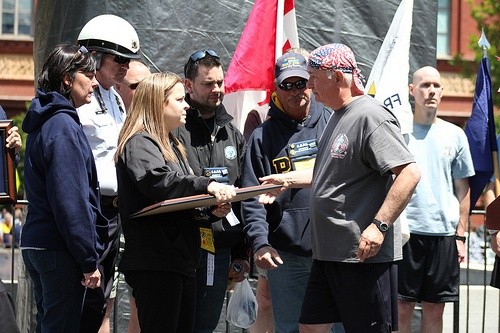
[97,195,118,207]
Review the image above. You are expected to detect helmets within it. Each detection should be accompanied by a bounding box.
[77,14,142,60]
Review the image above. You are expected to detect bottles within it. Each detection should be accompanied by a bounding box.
[226,263,242,293]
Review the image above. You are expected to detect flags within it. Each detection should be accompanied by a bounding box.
[220,0,301,135]
[363,0,414,135]
[461,55,497,214]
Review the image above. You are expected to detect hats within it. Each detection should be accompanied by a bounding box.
[274,53,310,85]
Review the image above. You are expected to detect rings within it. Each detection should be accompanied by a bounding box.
[221,193,226,196]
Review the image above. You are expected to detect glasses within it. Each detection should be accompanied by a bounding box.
[185,50,220,77]
[70,45,86,64]
[100,52,131,64]
[278,79,308,91]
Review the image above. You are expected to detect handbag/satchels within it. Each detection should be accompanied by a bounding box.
[227,272,257,328]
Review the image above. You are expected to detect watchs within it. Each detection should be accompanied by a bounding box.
[372,218,390,233]
[454,235,466,243]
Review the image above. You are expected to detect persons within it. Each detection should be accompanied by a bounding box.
[172,49,255,333]
[21,42,110,333]
[65,14,140,333]
[114,71,237,333]
[396,66,475,333]
[483,133,500,290]
[258,43,422,333]
[238,49,338,333]
[0,105,22,249]
[95,61,151,333]
